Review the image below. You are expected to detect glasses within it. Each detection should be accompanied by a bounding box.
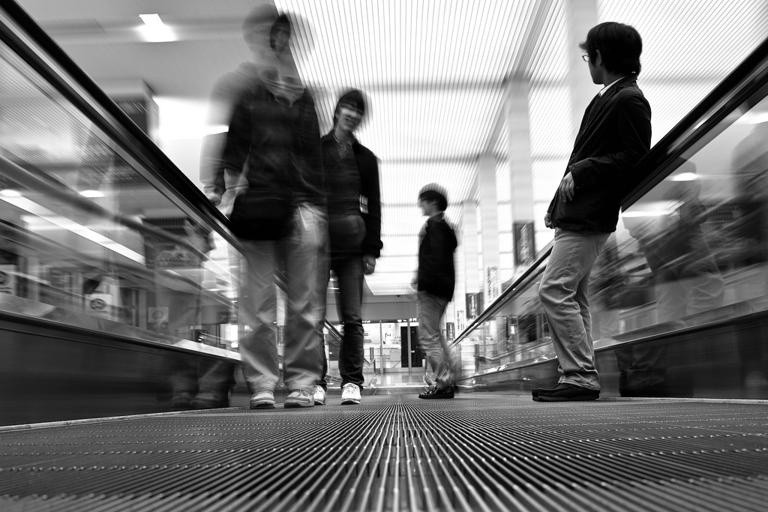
[582,54,590,61]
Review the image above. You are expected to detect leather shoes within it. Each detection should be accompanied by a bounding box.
[418,384,454,399]
[531,383,600,402]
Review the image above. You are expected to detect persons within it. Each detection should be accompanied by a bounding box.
[621,156,727,327]
[313,90,382,404]
[532,22,652,400]
[411,184,457,399]
[199,4,329,407]
[78,125,237,411]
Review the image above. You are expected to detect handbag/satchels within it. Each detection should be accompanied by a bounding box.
[233,189,294,240]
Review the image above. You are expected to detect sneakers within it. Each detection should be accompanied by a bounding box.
[313,385,325,404]
[284,390,314,408]
[340,383,361,404]
[249,390,275,408]
